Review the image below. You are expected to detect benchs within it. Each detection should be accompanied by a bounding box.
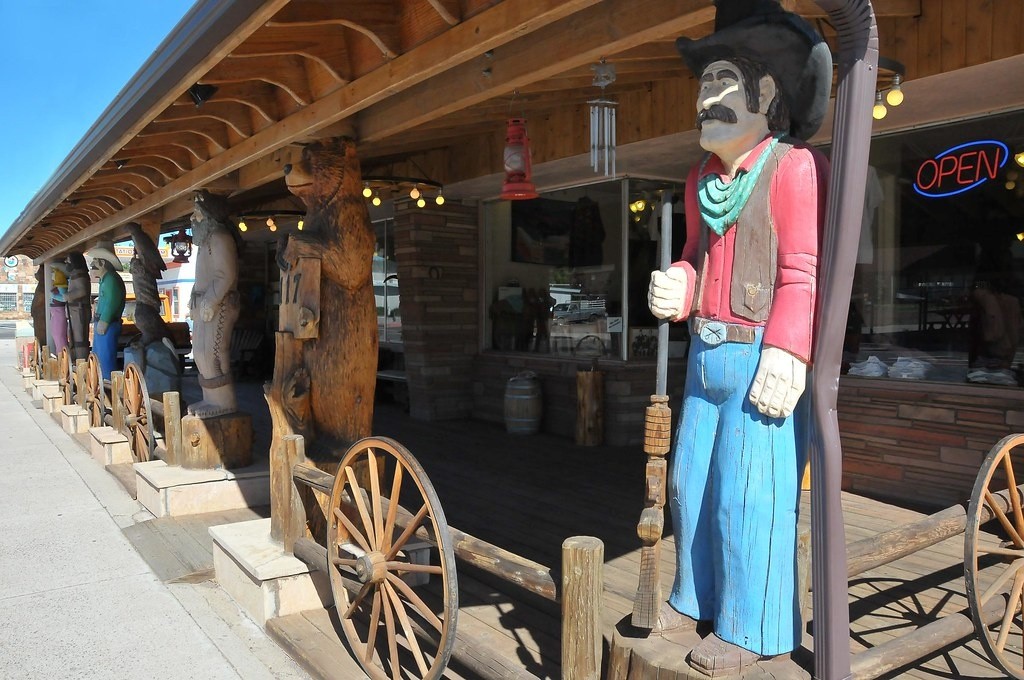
[377,369,408,412]
[115,322,191,374]
[191,328,265,379]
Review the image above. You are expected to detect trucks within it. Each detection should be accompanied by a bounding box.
[552,300,606,328]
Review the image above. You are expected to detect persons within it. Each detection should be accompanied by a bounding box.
[647,48,831,677]
[49,269,68,356]
[63,251,92,366]
[189,201,241,419]
[89,252,126,381]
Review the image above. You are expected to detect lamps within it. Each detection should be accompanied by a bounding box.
[163,228,193,264]
[362,155,445,208]
[499,94,540,200]
[237,195,309,233]
[815,16,906,119]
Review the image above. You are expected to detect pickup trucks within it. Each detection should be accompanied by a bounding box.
[87,292,194,374]
